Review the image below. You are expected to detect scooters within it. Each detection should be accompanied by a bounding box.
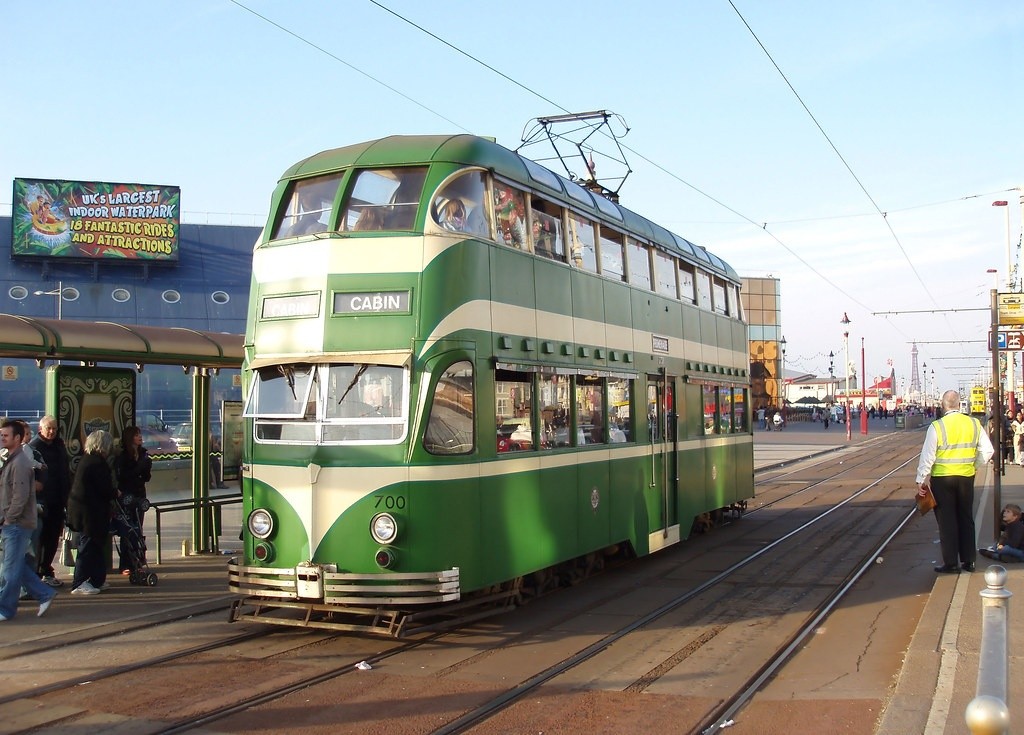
[773,420,784,431]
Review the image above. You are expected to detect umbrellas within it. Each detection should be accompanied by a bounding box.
[794,396,838,406]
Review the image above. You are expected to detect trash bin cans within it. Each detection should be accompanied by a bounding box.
[895,412,905,428]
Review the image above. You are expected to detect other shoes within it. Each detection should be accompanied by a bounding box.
[0,614,8,621]
[962,563,976,573]
[37,590,59,617]
[934,566,961,574]
[980,548,995,559]
[138,569,145,575]
[123,569,130,575]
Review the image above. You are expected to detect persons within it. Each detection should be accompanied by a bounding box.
[112,425,153,575]
[609,419,626,443]
[66,430,121,594]
[28,415,71,587]
[822,407,832,428]
[285,193,328,235]
[916,390,994,572]
[985,406,1024,467]
[893,407,902,418]
[511,417,547,441]
[0,417,58,621]
[773,412,784,424]
[906,406,941,420]
[866,405,888,420]
[764,406,773,430]
[550,408,585,444]
[439,199,489,236]
[980,504,1024,561]
[354,191,419,230]
[209,430,229,489]
[756,405,765,429]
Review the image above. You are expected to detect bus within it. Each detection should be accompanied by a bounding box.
[958,400,971,415]
[894,402,922,415]
[971,384,987,416]
[225,132,756,636]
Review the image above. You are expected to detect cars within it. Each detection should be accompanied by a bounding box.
[137,411,222,460]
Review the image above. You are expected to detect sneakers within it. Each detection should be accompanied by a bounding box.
[19,586,28,599]
[71,581,100,595]
[41,576,64,587]
[100,582,110,590]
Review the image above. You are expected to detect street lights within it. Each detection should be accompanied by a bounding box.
[829,350,835,406]
[839,311,851,441]
[779,335,788,407]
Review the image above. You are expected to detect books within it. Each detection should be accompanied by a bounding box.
[914,484,937,516]
